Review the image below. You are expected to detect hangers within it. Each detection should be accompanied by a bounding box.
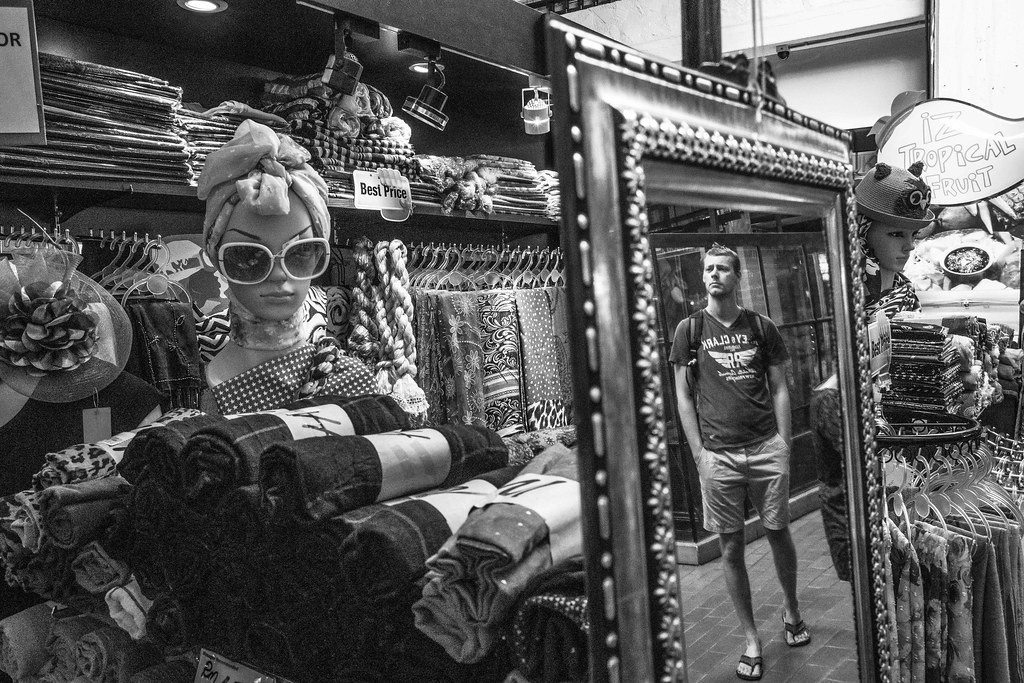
[4,231,193,310]
[884,441,1024,552]
[405,243,566,292]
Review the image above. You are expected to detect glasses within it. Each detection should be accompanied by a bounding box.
[216,237,331,285]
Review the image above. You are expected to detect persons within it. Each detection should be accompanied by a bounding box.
[667,241,811,680]
[853,158,936,325]
[0,247,163,498]
[163,117,381,414]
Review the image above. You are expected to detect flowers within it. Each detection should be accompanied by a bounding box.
[0,280,101,377]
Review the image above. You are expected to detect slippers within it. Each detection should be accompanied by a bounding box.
[782,610,811,647]
[735,654,763,680]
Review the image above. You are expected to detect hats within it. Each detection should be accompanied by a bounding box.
[854,160,935,230]
[0,249,132,403]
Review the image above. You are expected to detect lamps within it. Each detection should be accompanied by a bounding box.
[401,60,449,131]
[521,88,553,135]
[321,29,363,96]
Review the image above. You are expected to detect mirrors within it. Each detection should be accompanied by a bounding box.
[543,10,894,683]
